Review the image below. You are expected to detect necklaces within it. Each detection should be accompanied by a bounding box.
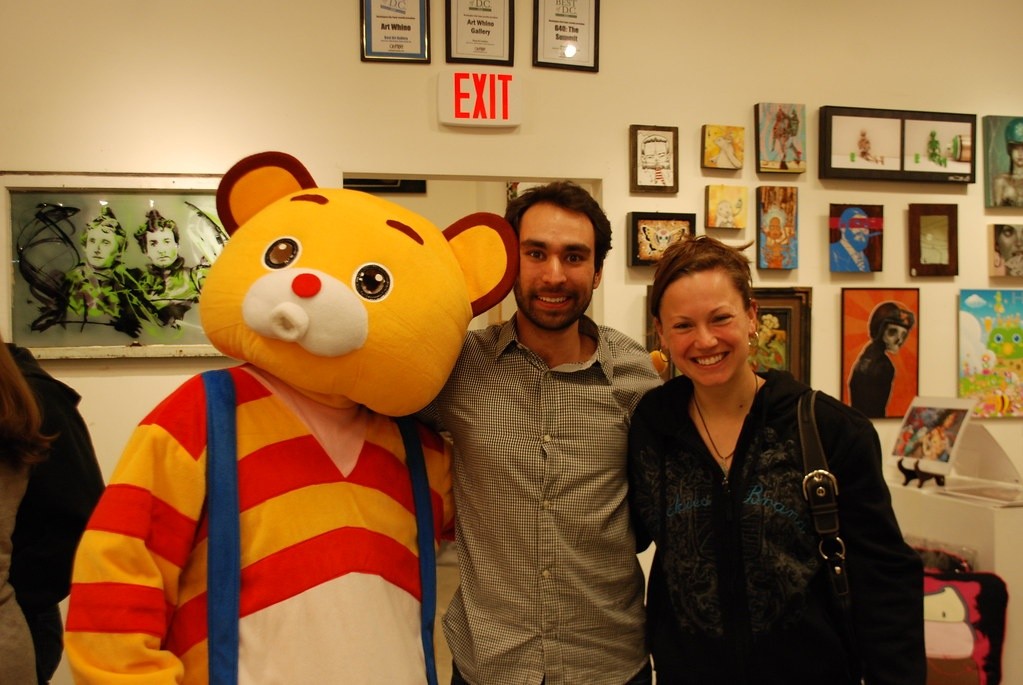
[692,369,758,479]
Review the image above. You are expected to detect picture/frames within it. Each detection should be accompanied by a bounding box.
[908,203,960,278]
[885,395,978,477]
[841,287,920,419]
[359,0,432,63]
[445,0,515,68]
[646,285,684,383]
[626,212,697,267]
[532,0,601,73]
[630,124,679,195]
[0,170,230,362]
[746,286,813,388]
[817,105,977,184]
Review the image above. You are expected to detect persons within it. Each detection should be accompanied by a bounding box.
[58,150,668,685]
[6,342,103,685]
[403,182,666,685]
[991,119,1023,209]
[0,333,37,685]
[994,224,1023,279]
[624,236,927,685]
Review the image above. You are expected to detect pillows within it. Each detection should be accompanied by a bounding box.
[924,571,1009,685]
